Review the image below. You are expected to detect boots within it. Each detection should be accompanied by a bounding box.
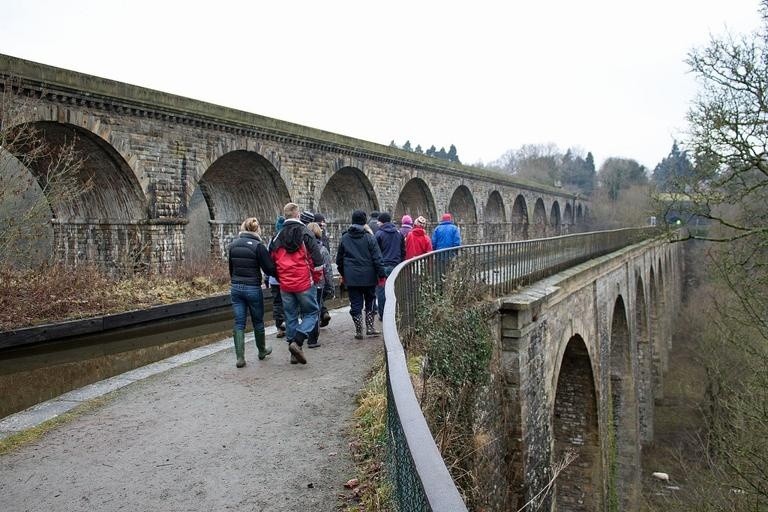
[253,331,273,360]
[365,314,380,335]
[351,312,364,340]
[232,329,247,368]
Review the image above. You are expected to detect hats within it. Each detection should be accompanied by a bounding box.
[370,212,377,218]
[351,210,367,224]
[441,214,451,222]
[401,216,412,225]
[276,217,285,232]
[414,216,427,228]
[378,213,391,223]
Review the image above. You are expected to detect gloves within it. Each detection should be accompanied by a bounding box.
[323,289,334,301]
[379,276,385,286]
[264,281,269,289]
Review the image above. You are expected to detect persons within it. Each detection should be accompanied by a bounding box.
[405,215,434,260]
[399,214,414,238]
[269,203,323,365]
[265,216,286,338]
[336,211,387,339]
[314,213,331,327]
[431,214,461,250]
[374,213,406,320]
[229,217,276,368]
[364,210,379,316]
[307,222,335,348]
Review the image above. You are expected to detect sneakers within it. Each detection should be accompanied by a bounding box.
[309,343,320,347]
[289,342,308,365]
[277,328,286,337]
[281,323,285,328]
[290,355,297,364]
[320,313,331,327]
